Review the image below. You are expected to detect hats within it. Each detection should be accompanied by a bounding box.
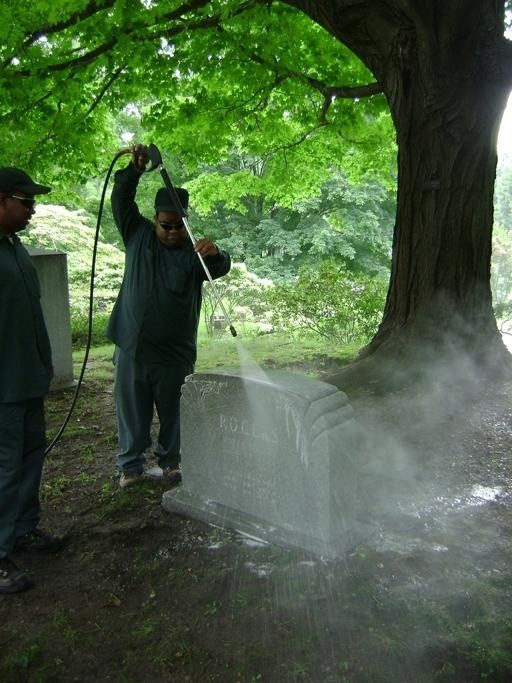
[154,187,189,213]
[0,167,51,195]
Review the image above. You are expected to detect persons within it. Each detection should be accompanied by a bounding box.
[1,163,76,595]
[107,142,231,488]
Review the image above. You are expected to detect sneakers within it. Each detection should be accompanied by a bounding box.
[119,471,143,494]
[14,525,60,550]
[161,466,182,487]
[0,565,32,592]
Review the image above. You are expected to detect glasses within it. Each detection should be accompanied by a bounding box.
[7,195,35,208]
[158,217,184,231]
